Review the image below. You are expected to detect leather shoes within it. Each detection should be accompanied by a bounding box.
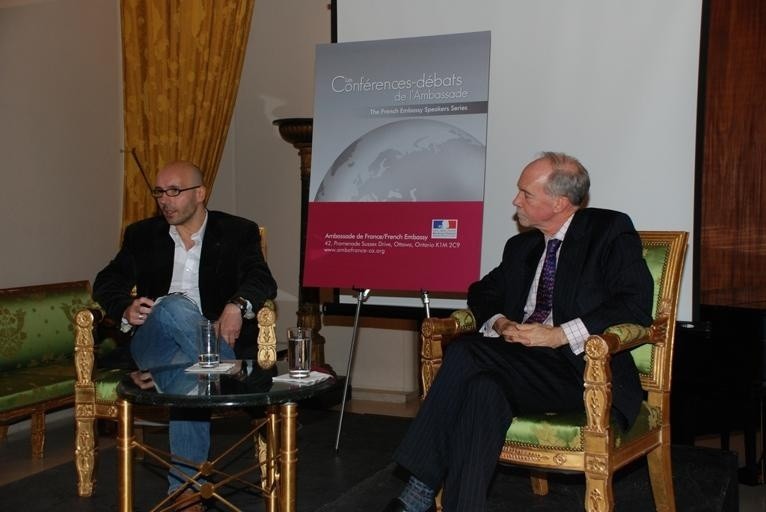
[381,497,437,512]
[169,484,207,512]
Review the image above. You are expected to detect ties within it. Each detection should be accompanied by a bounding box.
[523,238,563,325]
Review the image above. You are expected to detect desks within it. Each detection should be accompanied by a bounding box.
[114,366,338,509]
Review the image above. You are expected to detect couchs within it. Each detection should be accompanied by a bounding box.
[3,281,105,458]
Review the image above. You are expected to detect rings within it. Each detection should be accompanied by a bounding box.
[139,313,143,320]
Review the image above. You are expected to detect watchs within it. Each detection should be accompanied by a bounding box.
[226,295,247,316]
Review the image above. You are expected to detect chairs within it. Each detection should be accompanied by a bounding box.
[419,229,689,509]
[71,226,277,498]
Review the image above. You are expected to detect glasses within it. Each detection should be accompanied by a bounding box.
[150,185,201,199]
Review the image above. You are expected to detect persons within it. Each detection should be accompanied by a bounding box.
[94,158,276,511]
[386,150,655,512]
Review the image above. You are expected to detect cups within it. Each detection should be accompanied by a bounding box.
[197,320,222,369]
[199,371,220,396]
[287,328,312,379]
